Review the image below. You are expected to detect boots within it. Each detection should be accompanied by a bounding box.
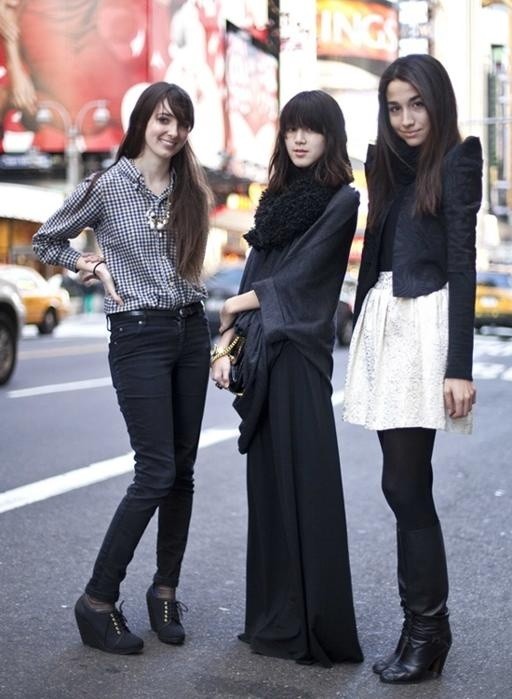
[380,521,452,685]
[370,526,410,675]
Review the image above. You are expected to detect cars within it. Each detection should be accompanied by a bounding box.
[204,268,356,346]
[0,265,70,385]
[474,271,512,328]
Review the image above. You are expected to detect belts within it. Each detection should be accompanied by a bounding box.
[111,303,202,325]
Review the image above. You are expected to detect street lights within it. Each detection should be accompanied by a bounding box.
[36,99,110,196]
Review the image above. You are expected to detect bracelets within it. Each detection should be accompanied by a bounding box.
[92,260,107,277]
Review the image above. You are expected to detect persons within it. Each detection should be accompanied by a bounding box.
[336,48,481,691]
[208,89,372,670]
[31,78,210,656]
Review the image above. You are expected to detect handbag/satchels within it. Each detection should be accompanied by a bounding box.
[210,336,247,393]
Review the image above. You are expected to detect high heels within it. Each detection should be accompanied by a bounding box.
[74,596,145,656]
[146,584,187,646]
